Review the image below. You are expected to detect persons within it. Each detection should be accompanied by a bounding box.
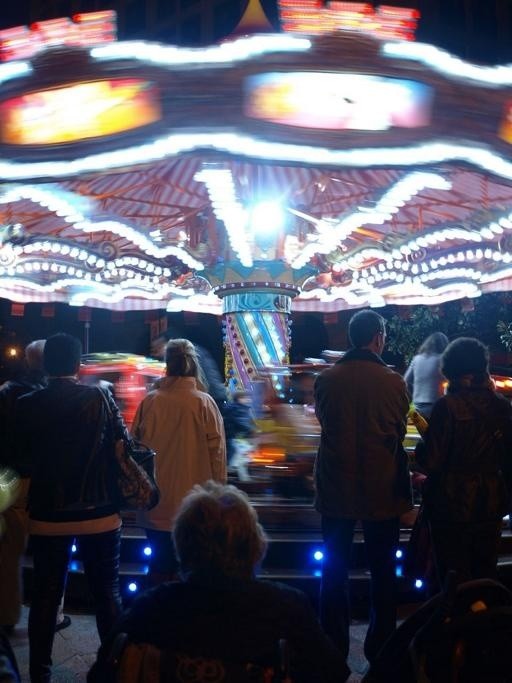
[126,337,230,594]
[14,332,135,680]
[309,308,412,668]
[412,335,510,593]
[0,338,73,680]
[86,478,354,682]
[400,330,450,424]
[150,331,254,468]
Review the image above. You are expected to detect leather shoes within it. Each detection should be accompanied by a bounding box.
[55,616,70,632]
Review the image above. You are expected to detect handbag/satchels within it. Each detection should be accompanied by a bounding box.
[113,439,160,520]
